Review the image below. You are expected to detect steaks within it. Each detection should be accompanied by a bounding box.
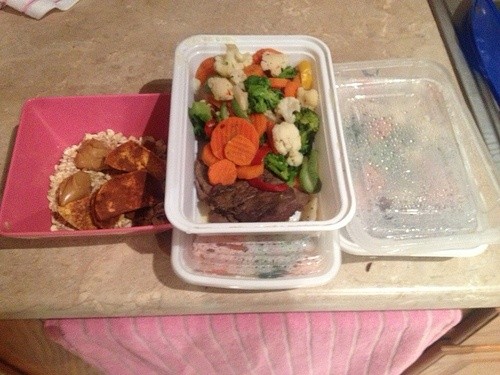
[194,163,311,223]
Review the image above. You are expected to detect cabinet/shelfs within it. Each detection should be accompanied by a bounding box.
[0,0,500,373]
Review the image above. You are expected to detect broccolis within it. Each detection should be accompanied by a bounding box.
[189,43,320,194]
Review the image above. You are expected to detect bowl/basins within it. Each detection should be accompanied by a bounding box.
[1,91,173,239]
